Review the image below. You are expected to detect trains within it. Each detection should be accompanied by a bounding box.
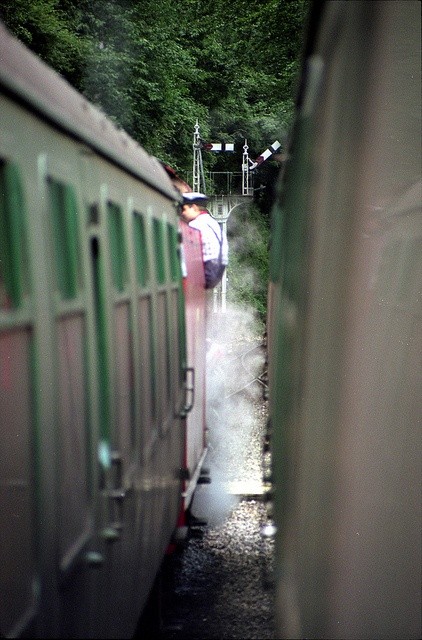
[0,24,210,640]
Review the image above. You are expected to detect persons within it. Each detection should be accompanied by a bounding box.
[181,190,230,289]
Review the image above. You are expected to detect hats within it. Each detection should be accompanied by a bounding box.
[182,192,208,205]
